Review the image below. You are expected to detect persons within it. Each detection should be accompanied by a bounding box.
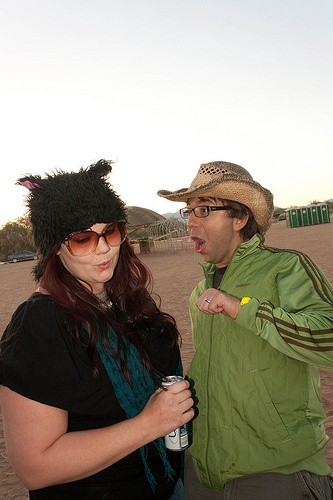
[157,161,333,500]
[0,160,197,500]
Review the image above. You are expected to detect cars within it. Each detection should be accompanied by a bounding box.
[278,213,286,221]
[7,249,39,263]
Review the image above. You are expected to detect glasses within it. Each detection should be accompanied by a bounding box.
[179,205,234,219]
[63,218,128,257]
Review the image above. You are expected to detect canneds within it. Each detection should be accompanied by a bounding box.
[161,376,189,451]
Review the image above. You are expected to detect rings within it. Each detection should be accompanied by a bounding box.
[204,298,211,305]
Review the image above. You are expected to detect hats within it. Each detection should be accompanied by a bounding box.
[15,160,125,279]
[156,162,273,237]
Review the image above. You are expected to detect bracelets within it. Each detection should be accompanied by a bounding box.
[240,296,251,306]
